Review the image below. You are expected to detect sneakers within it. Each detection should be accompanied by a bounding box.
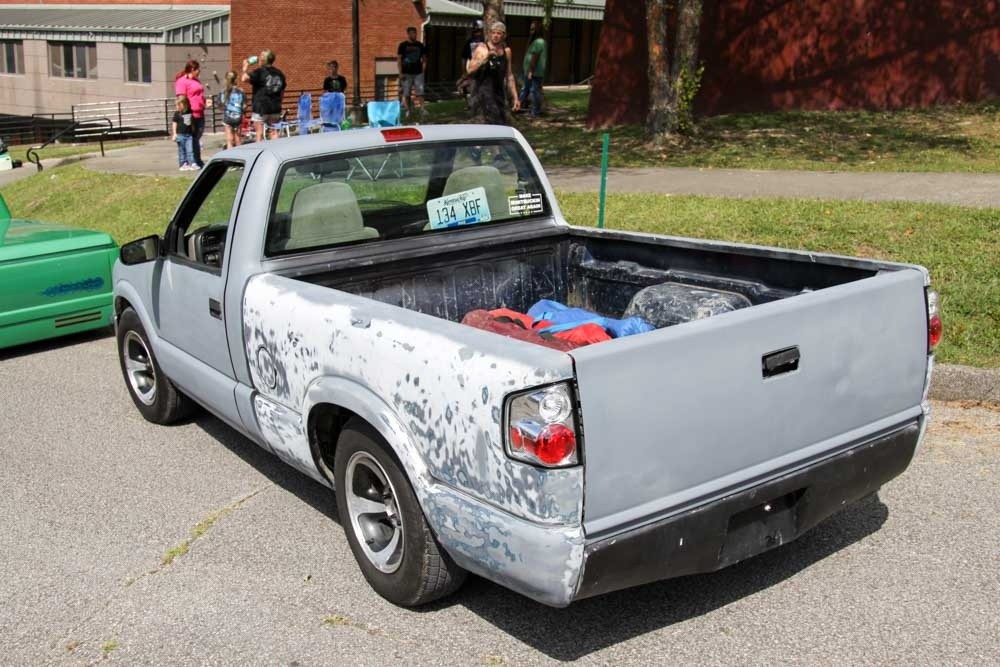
[190,163,201,170]
[179,162,190,171]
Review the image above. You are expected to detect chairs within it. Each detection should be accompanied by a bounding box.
[423,167,517,233]
[353,100,403,130]
[282,93,346,139]
[264,93,311,139]
[284,182,380,250]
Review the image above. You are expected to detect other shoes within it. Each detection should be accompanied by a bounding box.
[406,111,411,117]
[464,105,470,110]
[419,110,429,116]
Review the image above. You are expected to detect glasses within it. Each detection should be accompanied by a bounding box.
[491,32,501,35]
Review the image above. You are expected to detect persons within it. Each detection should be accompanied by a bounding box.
[519,21,549,118]
[397,27,431,119]
[241,47,288,142]
[175,60,207,169]
[455,18,484,111]
[321,61,347,93]
[214,70,248,150]
[468,21,522,169]
[172,95,201,171]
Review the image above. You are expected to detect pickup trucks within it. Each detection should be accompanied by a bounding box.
[111,123,942,608]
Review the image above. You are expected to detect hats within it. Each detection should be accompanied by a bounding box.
[490,21,507,33]
